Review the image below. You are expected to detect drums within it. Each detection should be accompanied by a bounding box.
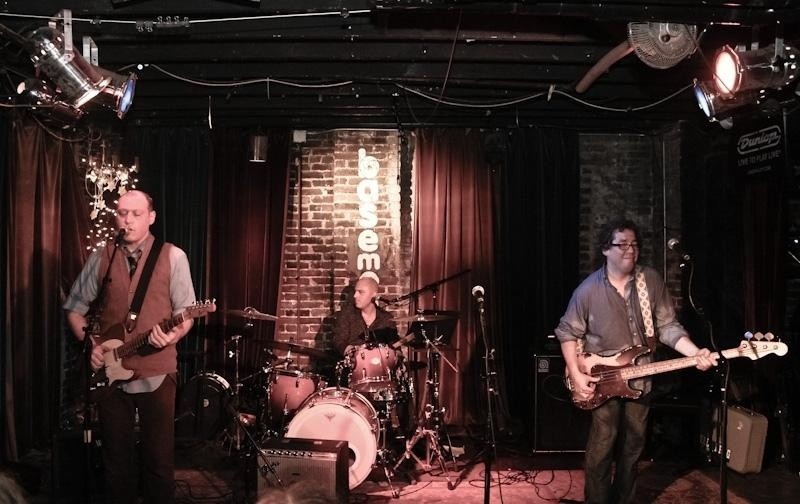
[348,343,404,394]
[184,369,235,424]
[282,385,383,492]
[255,369,328,422]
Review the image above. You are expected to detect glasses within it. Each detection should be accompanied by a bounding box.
[610,241,640,250]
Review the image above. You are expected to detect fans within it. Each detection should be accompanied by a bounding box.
[628,22,697,71]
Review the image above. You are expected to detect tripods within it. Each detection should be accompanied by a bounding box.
[195,338,245,457]
[377,348,455,499]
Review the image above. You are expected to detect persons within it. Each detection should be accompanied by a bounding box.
[62,189,197,503]
[331,277,402,360]
[554,219,718,503]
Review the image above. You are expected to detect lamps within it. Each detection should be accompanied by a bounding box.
[0,7,136,122]
[694,37,800,131]
[249,124,268,163]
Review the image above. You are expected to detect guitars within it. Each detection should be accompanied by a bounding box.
[74,298,219,405]
[564,331,789,411]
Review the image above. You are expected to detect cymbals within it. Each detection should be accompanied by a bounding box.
[222,309,279,322]
[258,337,336,365]
[412,342,462,352]
[388,314,455,323]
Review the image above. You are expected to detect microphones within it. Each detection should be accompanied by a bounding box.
[667,237,691,260]
[110,228,130,238]
[471,285,486,303]
